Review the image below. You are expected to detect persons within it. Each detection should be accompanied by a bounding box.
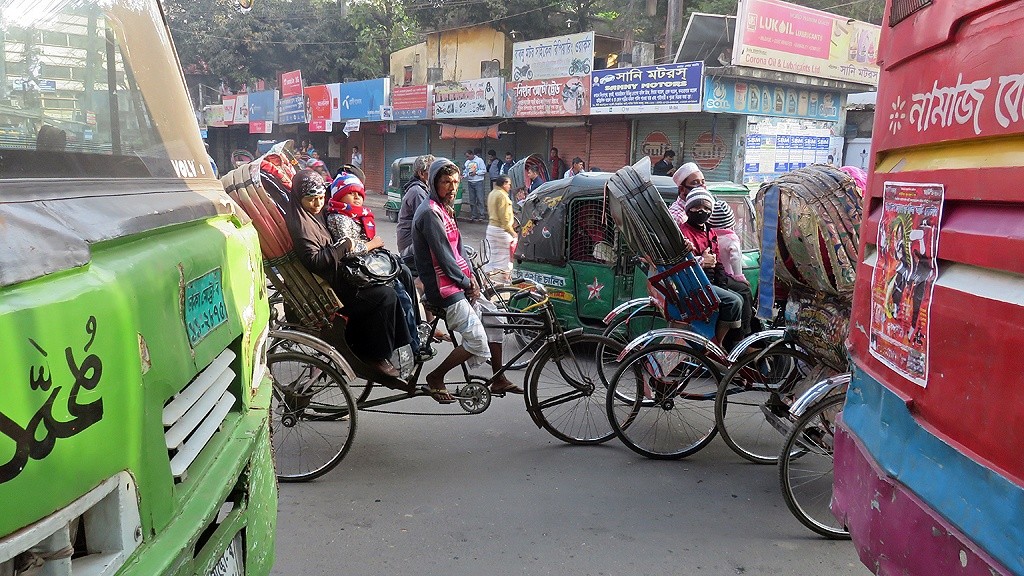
[668,163,761,363]
[294,141,332,181]
[485,82,496,116]
[460,150,487,220]
[564,158,600,178]
[304,94,313,122]
[548,148,564,180]
[411,160,524,403]
[396,155,451,343]
[352,146,362,169]
[285,170,422,377]
[483,174,518,286]
[486,149,503,190]
[653,150,675,176]
[526,166,543,194]
[500,152,515,174]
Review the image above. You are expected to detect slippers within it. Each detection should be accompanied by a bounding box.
[492,383,525,394]
[436,334,454,342]
[421,385,457,402]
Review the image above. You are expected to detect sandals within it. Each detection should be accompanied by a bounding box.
[415,351,433,362]
[419,347,437,356]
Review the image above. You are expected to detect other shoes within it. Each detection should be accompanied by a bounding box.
[378,359,399,377]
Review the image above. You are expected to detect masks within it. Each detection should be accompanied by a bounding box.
[688,209,711,224]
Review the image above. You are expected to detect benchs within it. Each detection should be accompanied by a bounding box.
[270,279,360,371]
[642,267,721,344]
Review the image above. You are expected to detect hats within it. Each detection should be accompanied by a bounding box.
[331,172,365,202]
[673,162,701,187]
[414,155,436,171]
[685,187,715,216]
[708,200,736,229]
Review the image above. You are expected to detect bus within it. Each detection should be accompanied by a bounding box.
[831,0,1024,576]
[0,0,282,576]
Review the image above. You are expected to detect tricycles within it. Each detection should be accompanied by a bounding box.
[600,152,866,542]
[215,148,643,482]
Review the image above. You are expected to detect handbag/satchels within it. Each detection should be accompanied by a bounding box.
[340,247,403,288]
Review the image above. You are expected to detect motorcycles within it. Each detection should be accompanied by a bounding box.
[507,165,768,353]
[384,153,467,224]
